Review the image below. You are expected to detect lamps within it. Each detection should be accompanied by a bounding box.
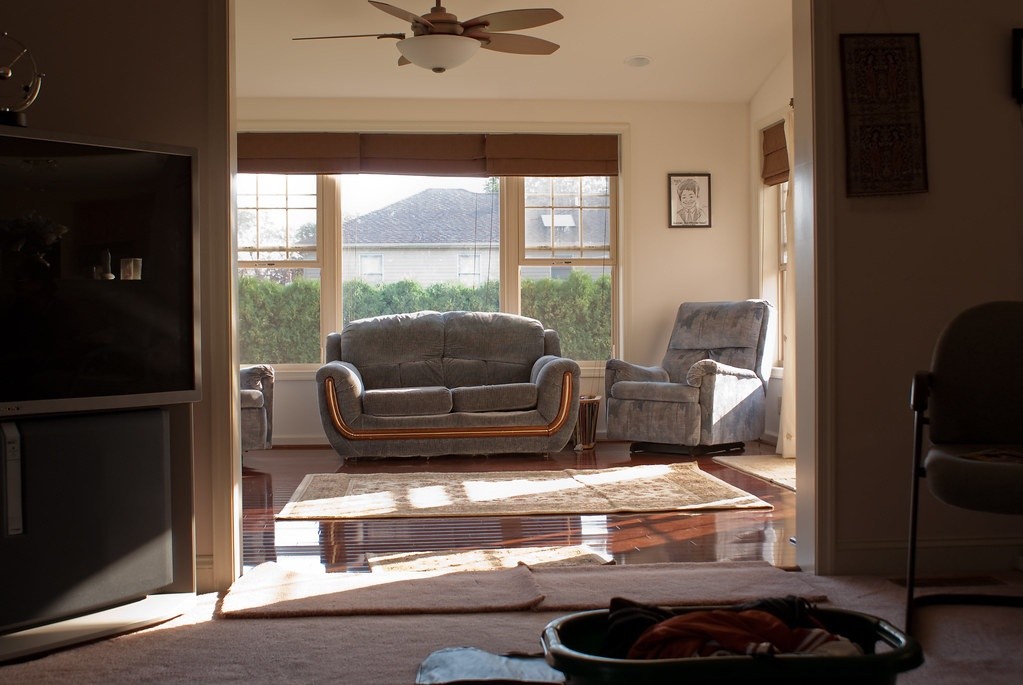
[395,35,481,73]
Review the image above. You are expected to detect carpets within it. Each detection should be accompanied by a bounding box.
[275,459,775,521]
[1,545,1023,684]
[714,457,795,492]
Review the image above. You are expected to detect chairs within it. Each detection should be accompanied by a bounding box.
[905,299,1023,634]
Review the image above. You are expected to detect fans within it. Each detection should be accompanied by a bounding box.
[292,0,564,55]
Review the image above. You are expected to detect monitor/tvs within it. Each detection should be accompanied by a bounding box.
[0,126,200,418]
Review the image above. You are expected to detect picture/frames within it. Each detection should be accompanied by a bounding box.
[668,173,711,228]
[837,32,929,196]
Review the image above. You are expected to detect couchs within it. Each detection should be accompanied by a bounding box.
[315,311,582,462]
[604,298,774,456]
[236,363,274,495]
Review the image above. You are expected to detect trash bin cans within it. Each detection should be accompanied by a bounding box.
[573,395,601,449]
